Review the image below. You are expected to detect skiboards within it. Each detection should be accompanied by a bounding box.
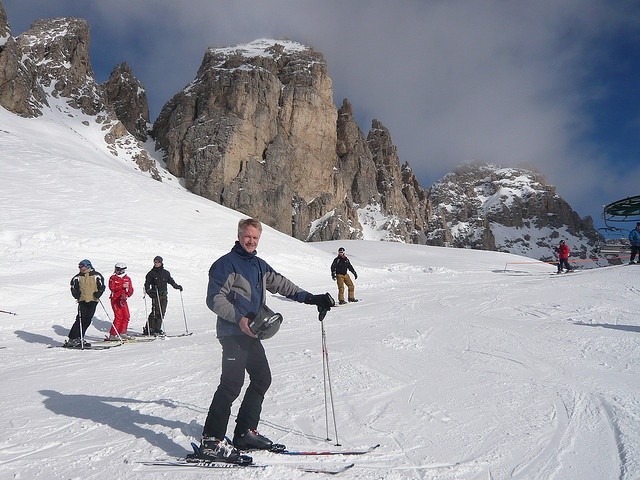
[142,333,193,337]
[104,339,155,342]
[168,444,380,474]
[58,344,120,349]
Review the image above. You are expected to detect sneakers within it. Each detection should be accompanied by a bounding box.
[110,334,131,340]
[233,428,272,449]
[348,298,358,302]
[339,300,347,305]
[143,327,163,336]
[200,433,240,461]
[67,338,91,347]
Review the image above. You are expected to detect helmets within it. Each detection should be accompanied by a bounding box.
[79,260,91,269]
[338,248,345,252]
[244,302,282,340]
[115,262,127,278]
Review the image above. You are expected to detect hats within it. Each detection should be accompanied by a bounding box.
[154,256,163,263]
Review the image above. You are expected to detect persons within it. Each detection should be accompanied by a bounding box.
[331,247,358,304]
[629,222,640,264]
[67,259,106,347]
[200,218,331,460]
[109,262,134,340]
[555,240,573,273]
[143,256,183,334]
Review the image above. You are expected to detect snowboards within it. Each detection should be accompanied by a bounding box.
[334,299,364,307]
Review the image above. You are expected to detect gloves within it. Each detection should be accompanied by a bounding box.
[331,272,337,281]
[304,293,330,310]
[149,289,156,298]
[173,284,183,291]
[74,291,80,299]
[119,293,129,307]
[317,292,335,321]
[93,290,103,298]
[352,271,357,280]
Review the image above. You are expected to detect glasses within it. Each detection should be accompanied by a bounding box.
[79,265,85,268]
